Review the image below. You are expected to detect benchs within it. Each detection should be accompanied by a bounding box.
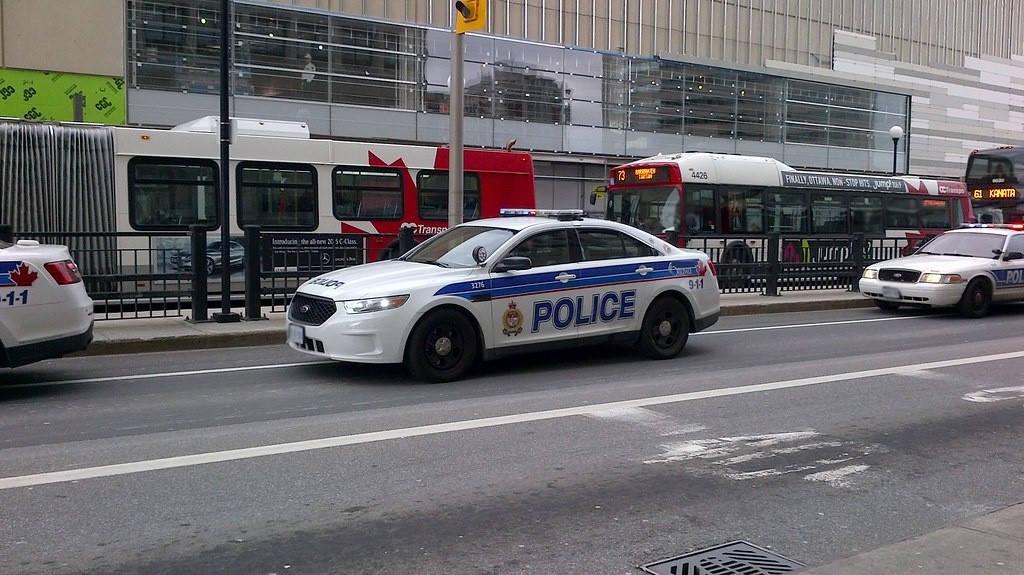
[585,245,641,257]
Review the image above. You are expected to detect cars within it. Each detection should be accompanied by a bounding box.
[858,222,1024,318]
[170,241,246,277]
[285,208,724,385]
[0,235,96,369]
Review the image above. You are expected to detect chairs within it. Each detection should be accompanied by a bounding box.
[348,198,479,220]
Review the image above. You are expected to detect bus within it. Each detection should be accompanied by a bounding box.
[589,151,975,288]
[965,145,1024,225]
[1,113,539,304]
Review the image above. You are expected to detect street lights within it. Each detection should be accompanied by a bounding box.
[890,125,903,176]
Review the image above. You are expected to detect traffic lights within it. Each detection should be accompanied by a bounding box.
[455,0,488,35]
